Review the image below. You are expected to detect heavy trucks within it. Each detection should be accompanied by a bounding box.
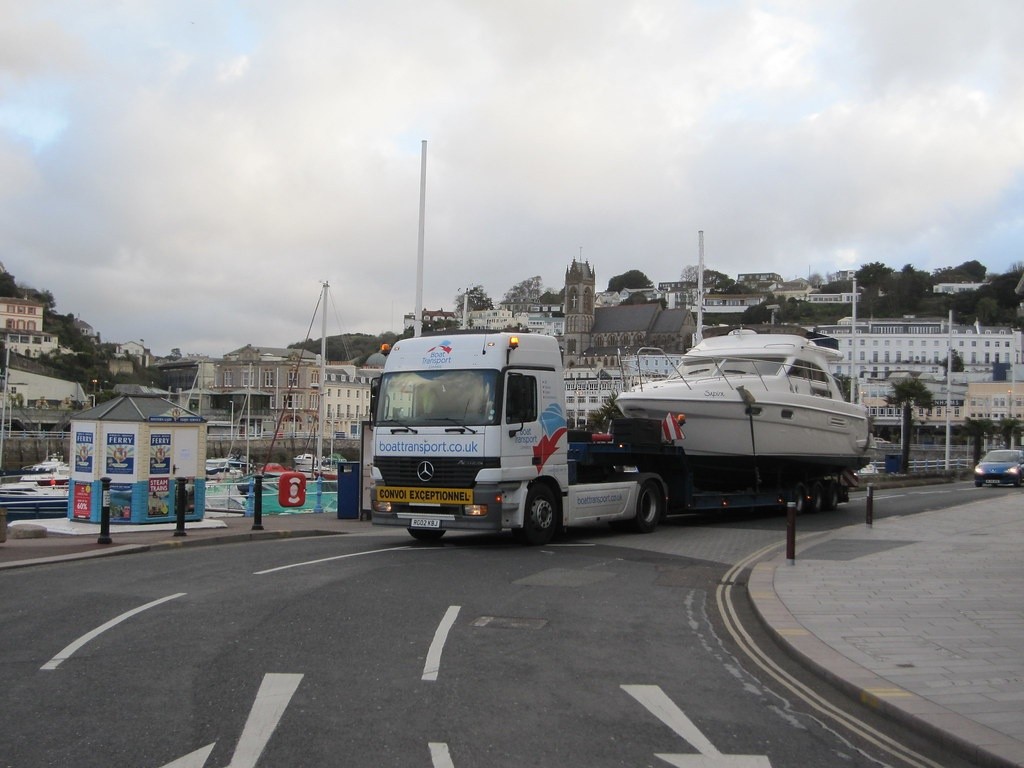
[369,331,861,546]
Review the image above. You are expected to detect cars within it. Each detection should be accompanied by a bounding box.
[974,449,1024,487]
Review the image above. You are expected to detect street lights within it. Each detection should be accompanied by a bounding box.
[88,394,95,408]
[92,380,97,393]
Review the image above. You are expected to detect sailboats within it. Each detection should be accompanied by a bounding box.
[176,281,362,517]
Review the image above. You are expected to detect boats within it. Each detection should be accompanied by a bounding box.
[293,454,317,465]
[614,323,877,467]
[0,452,69,510]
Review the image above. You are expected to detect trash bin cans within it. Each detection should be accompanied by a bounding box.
[885,453,904,474]
[337,462,359,520]
